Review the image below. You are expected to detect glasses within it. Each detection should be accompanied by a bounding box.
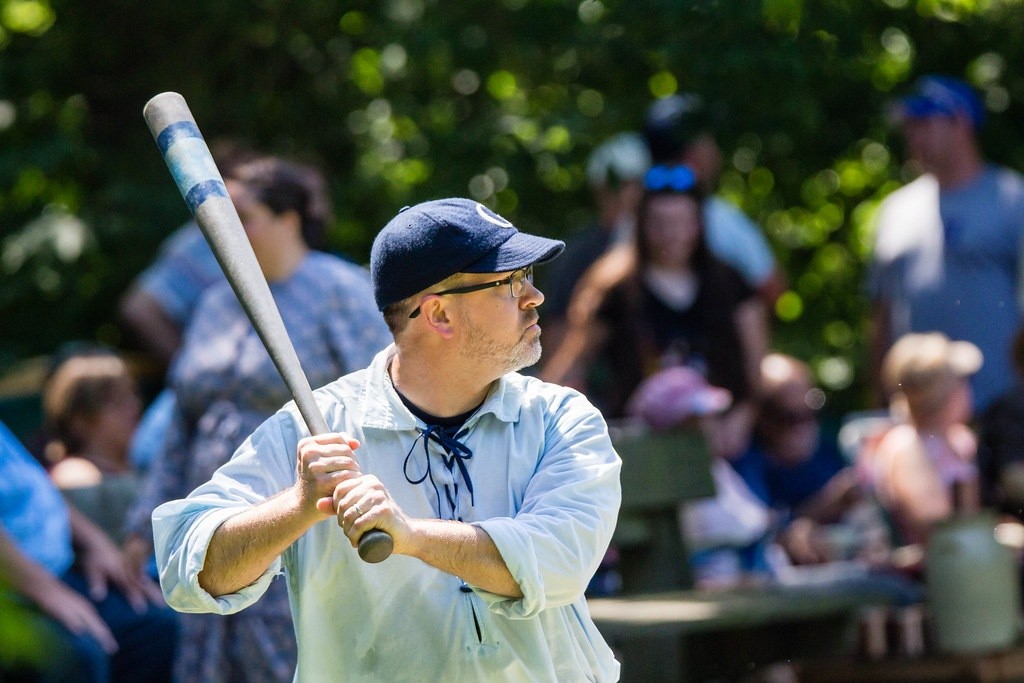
[409,266,533,319]
[639,165,697,191]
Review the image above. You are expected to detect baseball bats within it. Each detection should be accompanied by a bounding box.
[138,88,395,565]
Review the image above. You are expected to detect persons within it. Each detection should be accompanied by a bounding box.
[0,153,395,682]
[543,75,1024,682]
[153,197,622,683]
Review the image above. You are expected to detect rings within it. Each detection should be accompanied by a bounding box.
[354,504,363,516]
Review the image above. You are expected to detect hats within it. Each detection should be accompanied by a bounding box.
[884,334,983,391]
[901,75,979,121]
[589,133,650,190]
[370,198,565,311]
[649,94,706,129]
[636,368,733,425]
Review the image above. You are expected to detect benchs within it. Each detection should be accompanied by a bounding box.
[576,425,907,682]
